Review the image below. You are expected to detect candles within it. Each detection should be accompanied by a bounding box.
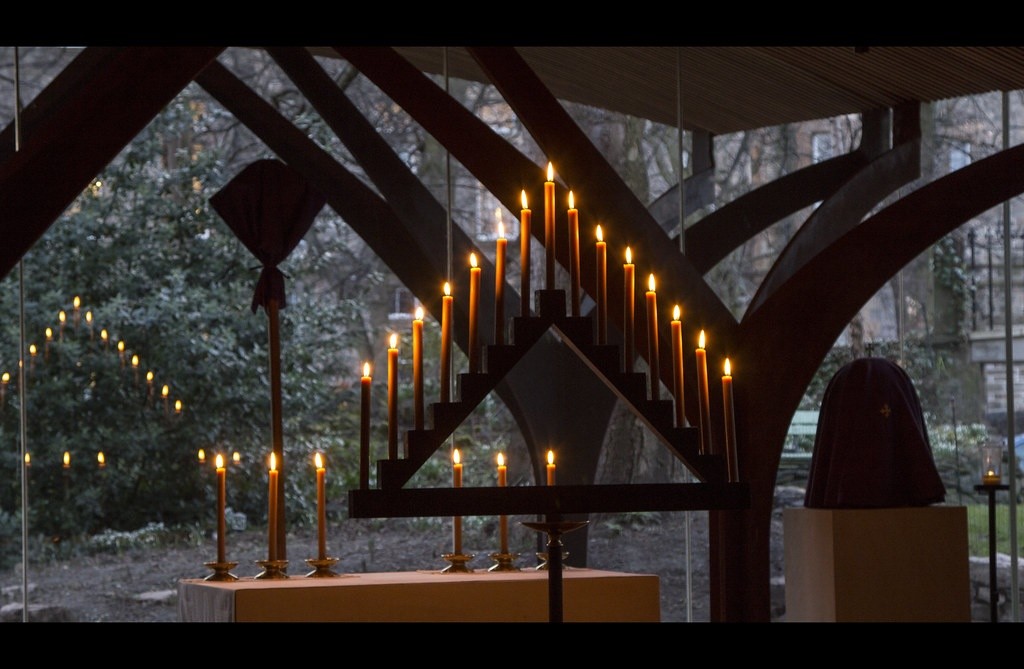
[693,329,712,454]
[544,162,554,290]
[439,282,453,403]
[386,333,400,459]
[412,307,424,431]
[453,448,462,556]
[0,296,207,469]
[721,359,738,482]
[669,304,685,427]
[268,451,278,561]
[623,247,633,372]
[232,451,241,465]
[359,362,372,490]
[214,453,226,564]
[493,221,507,344]
[496,451,509,554]
[520,190,532,317]
[983,470,998,484]
[469,252,482,374]
[646,273,659,400]
[595,224,606,344]
[544,451,557,522]
[566,190,580,316]
[314,453,326,559]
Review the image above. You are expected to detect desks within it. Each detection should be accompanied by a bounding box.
[177,563,660,621]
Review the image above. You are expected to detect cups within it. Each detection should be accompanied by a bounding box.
[977,435,1003,485]
